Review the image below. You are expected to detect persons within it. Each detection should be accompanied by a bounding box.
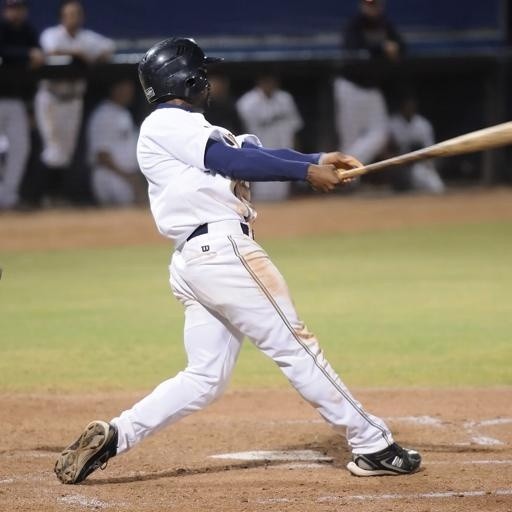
[333,1,406,166]
[52,36,423,486]
[234,67,307,198]
[26,1,116,203]
[1,1,42,206]
[388,96,448,191]
[85,78,144,205]
[207,68,246,136]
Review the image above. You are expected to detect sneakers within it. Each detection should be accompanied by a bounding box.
[345,441,421,478]
[53,420,117,483]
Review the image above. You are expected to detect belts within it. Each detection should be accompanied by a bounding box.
[187,218,255,240]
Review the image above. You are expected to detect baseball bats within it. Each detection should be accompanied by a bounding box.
[310,121,512,192]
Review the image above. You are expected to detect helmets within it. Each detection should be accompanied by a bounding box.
[136,38,226,104]
